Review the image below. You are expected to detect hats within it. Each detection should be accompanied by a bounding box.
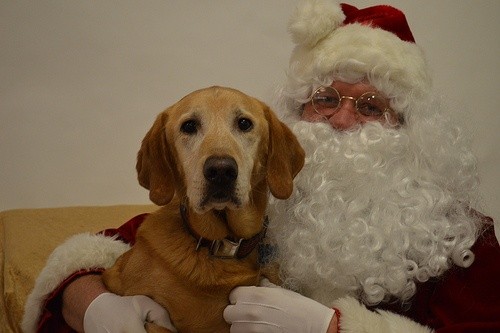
[277,3,435,103]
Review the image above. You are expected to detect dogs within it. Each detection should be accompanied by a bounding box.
[101,85,306,333]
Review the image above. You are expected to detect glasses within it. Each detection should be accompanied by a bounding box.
[307,85,397,123]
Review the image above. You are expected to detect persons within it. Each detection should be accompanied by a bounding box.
[20,0,500,333]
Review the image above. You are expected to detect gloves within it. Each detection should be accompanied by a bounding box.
[222,279,336,333]
[83,293,177,333]
